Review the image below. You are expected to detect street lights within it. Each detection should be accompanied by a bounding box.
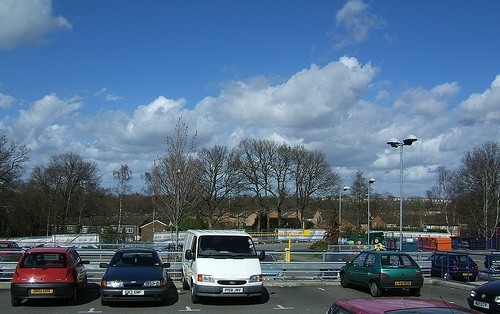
[339,186,351,250]
[368,178,375,245]
[386,134,418,252]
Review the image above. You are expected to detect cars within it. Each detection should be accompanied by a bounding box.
[326,296,486,314]
[11,247,91,308]
[425,253,479,282]
[467,279,500,314]
[99,248,171,306]
[0,241,19,247]
[339,250,423,297]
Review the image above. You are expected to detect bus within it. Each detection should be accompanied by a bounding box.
[366,230,452,251]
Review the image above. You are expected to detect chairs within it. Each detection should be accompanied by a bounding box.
[36,255,46,264]
[56,255,64,264]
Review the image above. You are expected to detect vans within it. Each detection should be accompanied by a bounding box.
[181,230,266,304]
